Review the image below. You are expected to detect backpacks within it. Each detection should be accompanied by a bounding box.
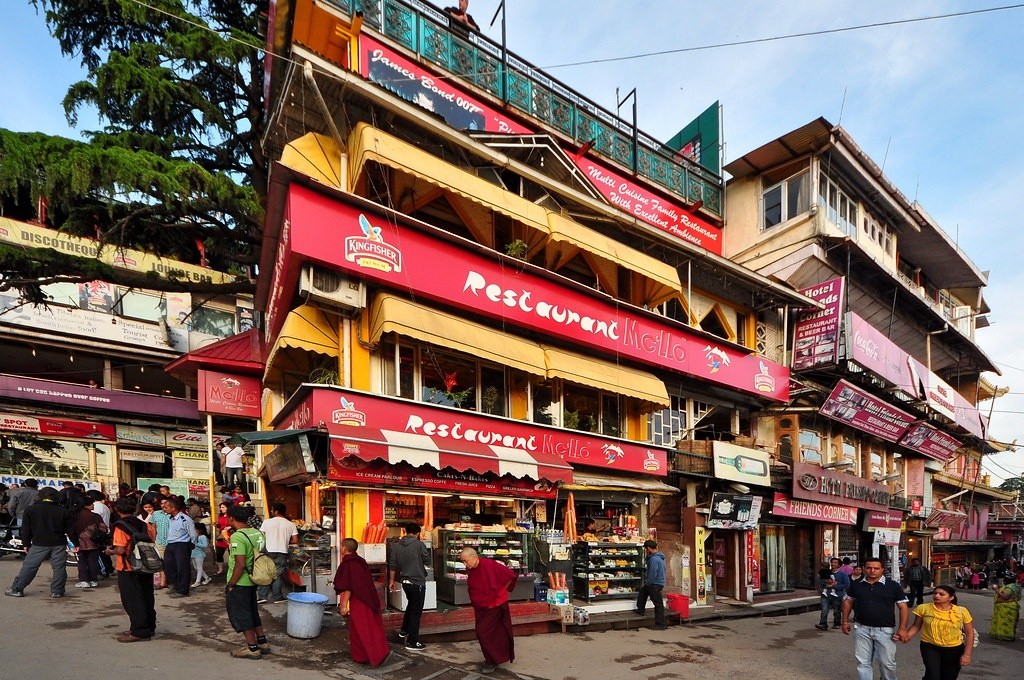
[114,520,164,573]
[230,531,278,586]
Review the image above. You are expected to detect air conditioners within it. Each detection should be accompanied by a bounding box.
[296,262,366,313]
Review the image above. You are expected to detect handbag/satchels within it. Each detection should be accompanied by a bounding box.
[89,530,106,545]
[959,606,979,648]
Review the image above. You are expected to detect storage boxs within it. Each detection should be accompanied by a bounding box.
[535,584,574,623]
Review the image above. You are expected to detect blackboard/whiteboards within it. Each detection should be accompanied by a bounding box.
[137,477,190,508]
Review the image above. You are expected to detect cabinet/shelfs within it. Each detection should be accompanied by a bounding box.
[572,541,645,600]
[442,531,533,577]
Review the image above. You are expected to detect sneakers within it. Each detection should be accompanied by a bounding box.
[52,592,62,597]
[5,589,23,597]
[75,581,90,587]
[90,580,98,587]
[256,642,270,654]
[230,645,262,660]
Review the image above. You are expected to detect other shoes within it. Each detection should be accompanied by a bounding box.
[166,589,184,597]
[274,598,287,603]
[191,583,200,586]
[257,598,267,603]
[117,635,151,642]
[398,631,407,638]
[406,643,425,650]
[822,592,828,597]
[482,661,494,673]
[123,630,155,636]
[202,577,211,584]
[820,625,827,630]
[830,592,838,598]
[833,624,839,629]
[633,609,644,616]
[652,625,666,630]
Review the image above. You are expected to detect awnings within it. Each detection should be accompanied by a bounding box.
[261,121,681,416]
[326,423,574,486]
[225,428,317,445]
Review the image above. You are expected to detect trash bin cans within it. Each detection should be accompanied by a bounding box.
[665,594,690,618]
[286,592,328,637]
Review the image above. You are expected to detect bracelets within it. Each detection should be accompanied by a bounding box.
[603,525,606,529]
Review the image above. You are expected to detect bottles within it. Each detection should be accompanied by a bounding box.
[576,507,628,528]
[719,455,768,477]
[539,529,569,543]
[517,520,534,533]
[574,605,590,625]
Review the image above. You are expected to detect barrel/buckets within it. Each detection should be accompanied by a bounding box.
[286,592,329,639]
[666,594,690,619]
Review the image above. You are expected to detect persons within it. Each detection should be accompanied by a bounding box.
[842,558,911,680]
[105,498,156,642]
[220,438,250,493]
[460,547,517,673]
[815,557,1022,641]
[0,478,299,604]
[415,512,425,536]
[891,585,974,680]
[634,540,665,630]
[582,518,608,535]
[333,538,394,667]
[389,523,431,650]
[225,505,271,660]
[212,443,226,485]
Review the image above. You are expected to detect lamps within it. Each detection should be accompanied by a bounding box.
[875,473,901,483]
[822,460,853,470]
